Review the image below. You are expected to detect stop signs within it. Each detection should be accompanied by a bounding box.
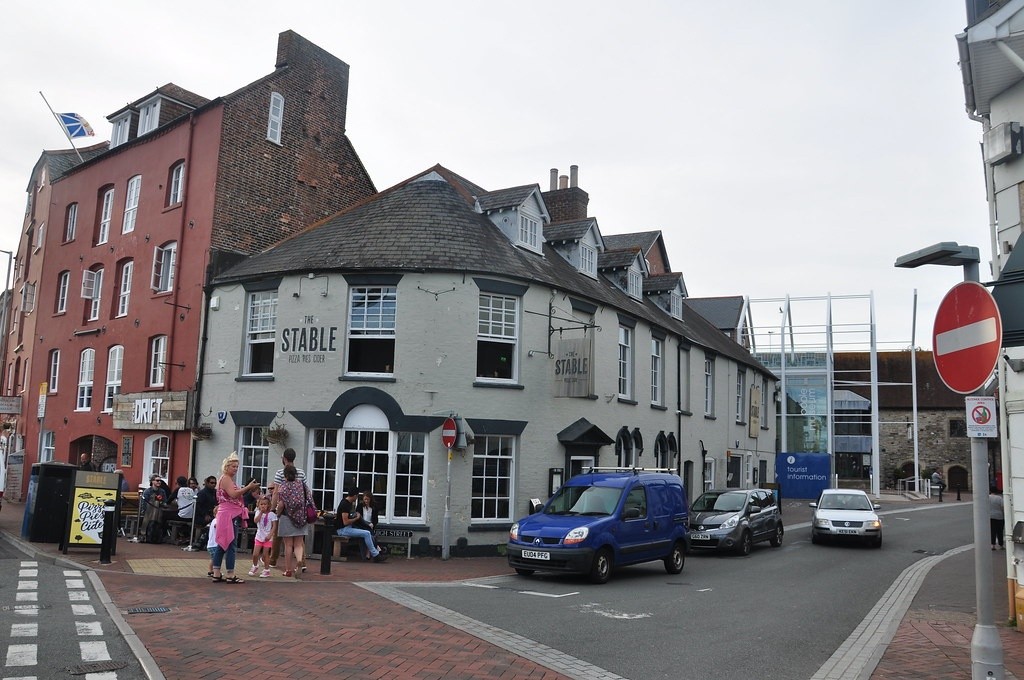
[931,282,1003,393]
[442,418,456,448]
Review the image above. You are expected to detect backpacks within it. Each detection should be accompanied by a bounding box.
[145,519,165,544]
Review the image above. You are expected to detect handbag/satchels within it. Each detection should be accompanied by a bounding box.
[303,482,317,524]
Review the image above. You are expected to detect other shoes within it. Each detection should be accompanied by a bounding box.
[366,551,371,560]
[207,571,224,577]
[294,563,307,572]
[260,556,277,568]
[377,545,384,554]
[374,554,390,562]
[282,570,292,577]
[294,561,302,578]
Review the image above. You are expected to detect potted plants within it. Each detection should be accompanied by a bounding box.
[264,421,287,448]
[191,426,212,441]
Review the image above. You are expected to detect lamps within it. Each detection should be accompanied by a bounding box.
[983,121,1024,166]
[292,292,300,298]
[320,291,327,297]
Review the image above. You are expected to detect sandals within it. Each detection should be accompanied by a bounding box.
[226,576,245,584]
[212,573,227,583]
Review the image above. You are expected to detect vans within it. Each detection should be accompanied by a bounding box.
[505,467,688,585]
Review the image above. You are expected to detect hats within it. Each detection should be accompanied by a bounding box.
[114,469,124,474]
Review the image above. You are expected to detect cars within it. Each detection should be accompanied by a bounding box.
[809,489,882,549]
[688,488,785,557]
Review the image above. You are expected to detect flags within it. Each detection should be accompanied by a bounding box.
[57,111,96,139]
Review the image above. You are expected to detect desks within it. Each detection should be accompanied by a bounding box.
[301,516,336,559]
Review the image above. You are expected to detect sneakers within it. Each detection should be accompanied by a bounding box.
[259,569,270,578]
[248,565,259,575]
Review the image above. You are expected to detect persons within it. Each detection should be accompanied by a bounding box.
[932,467,947,492]
[989,486,1006,550]
[79,453,96,472]
[116,448,390,584]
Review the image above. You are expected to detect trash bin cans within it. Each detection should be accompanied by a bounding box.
[21,461,81,541]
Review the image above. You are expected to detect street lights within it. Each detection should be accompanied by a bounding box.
[895,241,1004,680]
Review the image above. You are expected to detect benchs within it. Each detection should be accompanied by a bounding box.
[119,507,348,563]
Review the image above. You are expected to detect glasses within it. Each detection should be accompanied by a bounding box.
[268,487,274,490]
[156,480,162,482]
[209,482,217,485]
[190,483,196,486]
